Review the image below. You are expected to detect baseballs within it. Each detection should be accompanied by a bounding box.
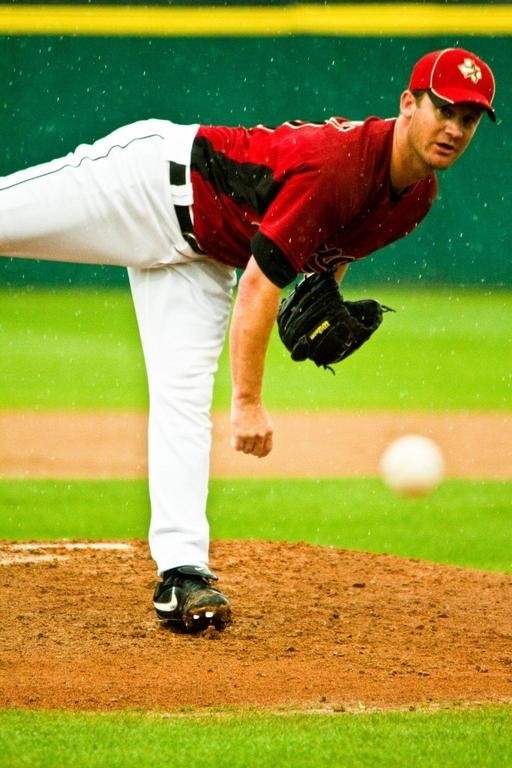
[378,437,442,496]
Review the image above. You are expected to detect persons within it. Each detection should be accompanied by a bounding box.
[0,45,498,635]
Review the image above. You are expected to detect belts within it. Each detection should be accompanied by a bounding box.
[169,162,207,255]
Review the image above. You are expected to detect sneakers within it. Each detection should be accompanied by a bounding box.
[152,566,231,632]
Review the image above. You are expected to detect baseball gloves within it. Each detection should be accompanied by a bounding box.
[276,267,385,368]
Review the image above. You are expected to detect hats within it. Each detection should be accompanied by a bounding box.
[408,48,496,122]
